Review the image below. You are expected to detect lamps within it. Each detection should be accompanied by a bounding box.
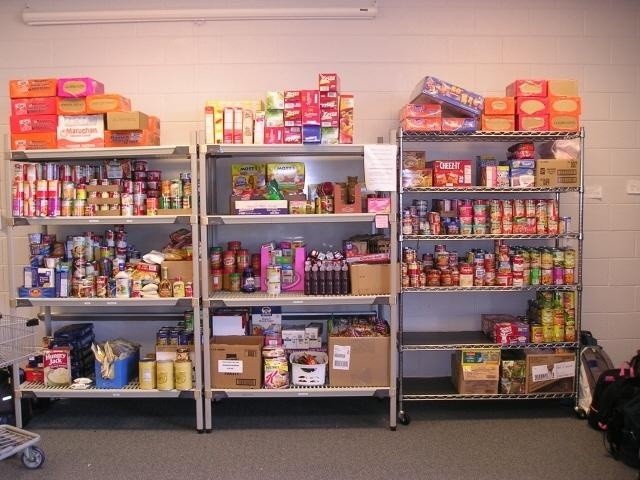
[21,4,379,24]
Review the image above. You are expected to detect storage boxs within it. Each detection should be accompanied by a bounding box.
[350,262,391,294]
[535,159,580,187]
[455,349,501,365]
[5,76,160,153]
[395,75,584,134]
[451,356,499,395]
[160,260,193,299]
[525,348,577,394]
[481,313,529,343]
[327,330,391,387]
[205,72,357,146]
[95,343,140,388]
[210,335,265,389]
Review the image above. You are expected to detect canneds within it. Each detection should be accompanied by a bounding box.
[210,241,282,294]
[138,310,194,391]
[74,225,141,298]
[402,196,575,342]
[12,159,192,217]
[315,197,334,214]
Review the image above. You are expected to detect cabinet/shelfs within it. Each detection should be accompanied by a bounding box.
[198,124,400,434]
[399,126,586,424]
[4,128,204,432]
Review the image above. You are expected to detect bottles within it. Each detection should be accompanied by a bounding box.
[171,275,185,297]
[114,262,131,298]
[303,260,349,296]
[158,266,172,297]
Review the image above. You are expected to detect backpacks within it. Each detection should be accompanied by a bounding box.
[577,329,640,470]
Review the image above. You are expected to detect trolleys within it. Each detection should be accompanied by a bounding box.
[0,314,46,470]
